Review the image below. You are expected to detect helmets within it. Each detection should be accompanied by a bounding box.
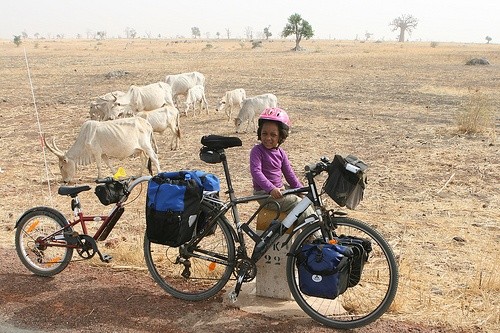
[258,107,290,131]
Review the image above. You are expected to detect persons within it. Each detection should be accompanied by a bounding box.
[250,107,322,241]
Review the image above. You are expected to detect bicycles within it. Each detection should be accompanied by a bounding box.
[13,143,163,277]
[143,132,401,331]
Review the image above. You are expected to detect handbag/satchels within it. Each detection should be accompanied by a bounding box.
[146,169,220,247]
[323,154,369,210]
[297,235,372,298]
[94,185,128,206]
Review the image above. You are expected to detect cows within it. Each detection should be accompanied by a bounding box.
[216,88,277,133]
[89,81,182,156]
[44,117,161,185]
[165,71,209,117]
